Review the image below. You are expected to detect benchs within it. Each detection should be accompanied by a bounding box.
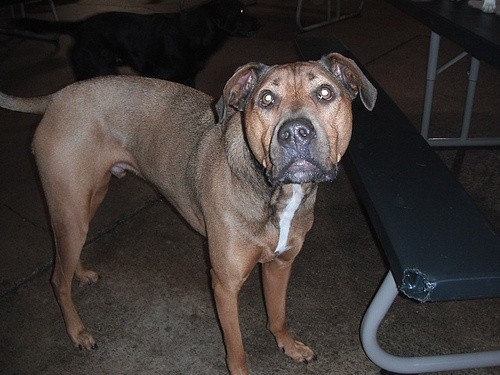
[297,0,500,148]
[298,37,500,374]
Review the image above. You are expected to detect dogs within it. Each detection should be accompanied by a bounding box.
[0,52,377,375]
[0,0,263,90]
